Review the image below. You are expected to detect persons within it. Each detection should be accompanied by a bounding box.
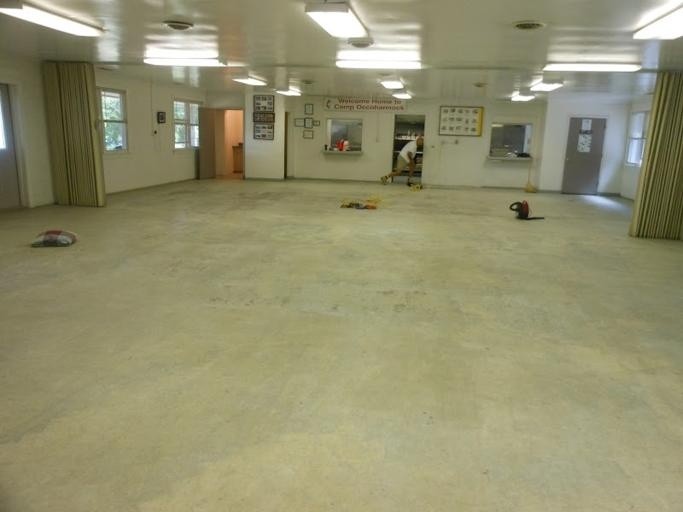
[380,136,422,188]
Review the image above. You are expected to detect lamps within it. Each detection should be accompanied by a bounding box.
[306,2,369,42]
[510,78,565,102]
[378,75,415,100]
[0,0,104,38]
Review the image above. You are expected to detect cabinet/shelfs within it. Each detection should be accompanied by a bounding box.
[394,135,423,171]
